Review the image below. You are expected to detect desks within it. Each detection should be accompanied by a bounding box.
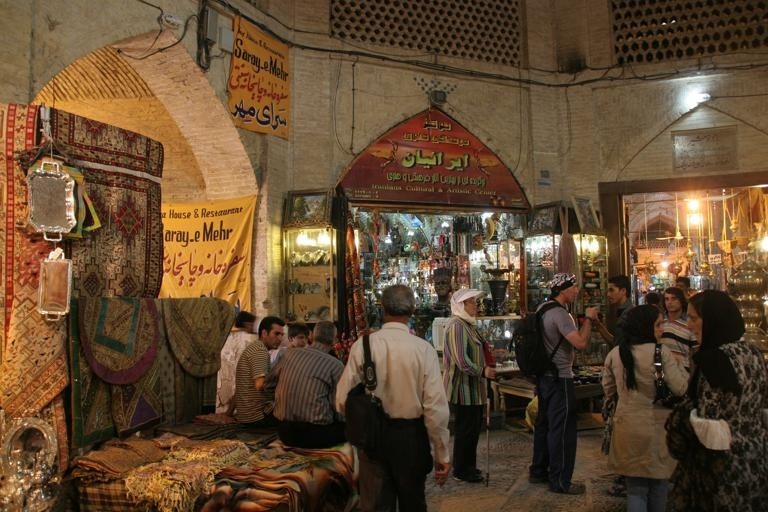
[489,375,606,434]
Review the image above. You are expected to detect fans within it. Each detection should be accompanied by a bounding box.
[656,191,709,240]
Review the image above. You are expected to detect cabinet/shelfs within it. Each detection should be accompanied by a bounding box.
[727,275,767,351]
[523,207,612,364]
[468,314,522,375]
[280,190,341,330]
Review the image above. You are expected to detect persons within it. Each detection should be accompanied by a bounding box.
[662,290,767,512]
[434,268,453,308]
[234,312,256,333]
[530,273,597,494]
[235,315,284,428]
[600,305,689,512]
[270,323,309,368]
[676,277,691,303]
[442,288,495,482]
[659,287,700,374]
[639,290,649,305]
[336,283,449,512]
[645,292,658,305]
[591,275,634,498]
[261,320,347,449]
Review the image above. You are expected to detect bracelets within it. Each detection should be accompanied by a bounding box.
[584,316,593,322]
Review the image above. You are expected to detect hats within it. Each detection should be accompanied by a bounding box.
[451,286,485,303]
[551,272,576,291]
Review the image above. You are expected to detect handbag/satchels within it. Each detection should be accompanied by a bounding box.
[665,414,700,463]
[655,380,687,407]
[482,342,496,367]
[345,391,384,450]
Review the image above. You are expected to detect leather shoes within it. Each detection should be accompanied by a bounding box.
[453,467,483,484]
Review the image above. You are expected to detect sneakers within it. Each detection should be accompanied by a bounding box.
[551,483,587,494]
[530,476,549,483]
[607,484,627,497]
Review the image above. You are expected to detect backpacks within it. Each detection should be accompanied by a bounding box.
[510,302,564,376]
